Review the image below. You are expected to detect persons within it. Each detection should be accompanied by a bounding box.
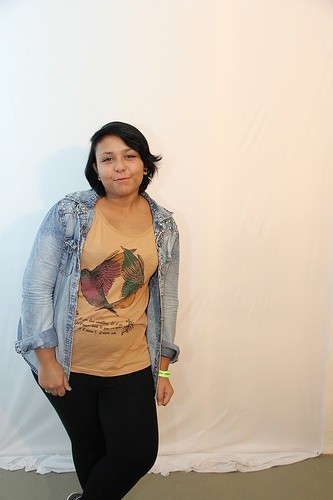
[14,121,181,500]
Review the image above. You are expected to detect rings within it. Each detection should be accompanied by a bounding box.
[45,389,51,393]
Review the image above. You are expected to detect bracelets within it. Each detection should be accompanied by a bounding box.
[158,370,170,379]
